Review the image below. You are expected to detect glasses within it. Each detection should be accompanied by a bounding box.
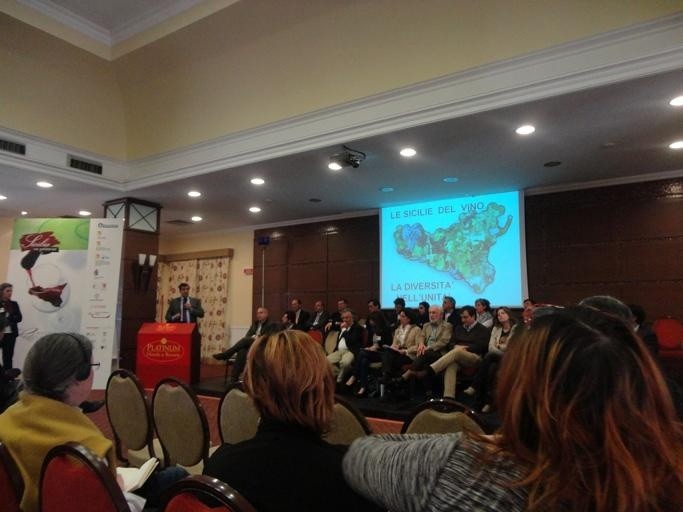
[522,304,565,329]
[87,361,101,372]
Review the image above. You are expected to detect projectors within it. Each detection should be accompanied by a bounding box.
[330,152,362,168]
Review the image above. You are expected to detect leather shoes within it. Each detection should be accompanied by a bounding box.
[213,353,229,360]
[464,387,476,395]
[483,405,491,413]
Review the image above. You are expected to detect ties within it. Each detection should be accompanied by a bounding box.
[340,328,348,339]
[183,298,187,323]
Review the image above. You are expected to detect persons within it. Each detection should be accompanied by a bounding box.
[0,282,21,370]
[197,326,387,510]
[0,364,106,415]
[630,304,659,358]
[0,332,122,511]
[340,302,682,509]
[165,280,205,325]
[214,296,538,417]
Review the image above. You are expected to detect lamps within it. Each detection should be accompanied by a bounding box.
[135,252,157,296]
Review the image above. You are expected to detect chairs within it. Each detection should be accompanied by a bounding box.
[653,317,683,364]
